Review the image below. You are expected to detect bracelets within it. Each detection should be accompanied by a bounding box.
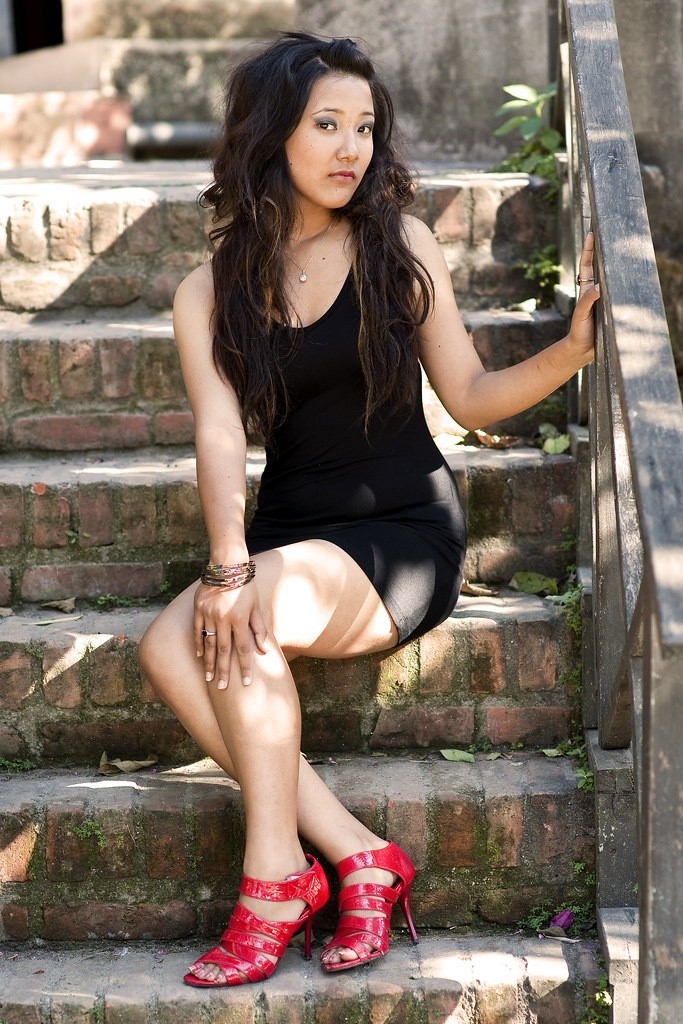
[200,560,256,588]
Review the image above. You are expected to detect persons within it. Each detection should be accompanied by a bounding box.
[138,29,599,990]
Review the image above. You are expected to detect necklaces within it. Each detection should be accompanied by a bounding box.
[283,214,337,282]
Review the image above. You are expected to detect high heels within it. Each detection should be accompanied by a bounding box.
[321,840,418,971]
[182,853,328,986]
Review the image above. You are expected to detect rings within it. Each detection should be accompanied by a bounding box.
[202,630,216,639]
[576,274,594,286]
